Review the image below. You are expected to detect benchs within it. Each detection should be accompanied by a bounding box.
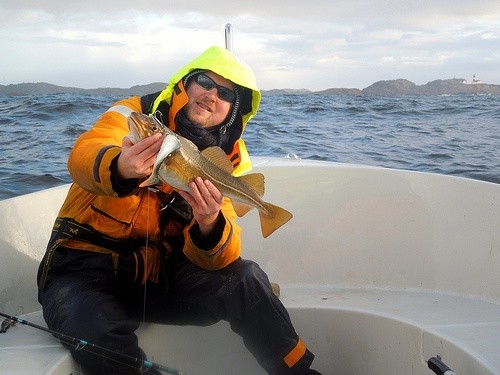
[1,287,499,374]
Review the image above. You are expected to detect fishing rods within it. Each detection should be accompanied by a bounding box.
[0,311,187,375]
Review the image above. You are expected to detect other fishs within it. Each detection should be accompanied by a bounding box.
[127,112,293,239]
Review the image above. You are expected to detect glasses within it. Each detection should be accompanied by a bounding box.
[193,73,236,104]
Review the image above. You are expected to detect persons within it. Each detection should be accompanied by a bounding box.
[36,44,321,375]
